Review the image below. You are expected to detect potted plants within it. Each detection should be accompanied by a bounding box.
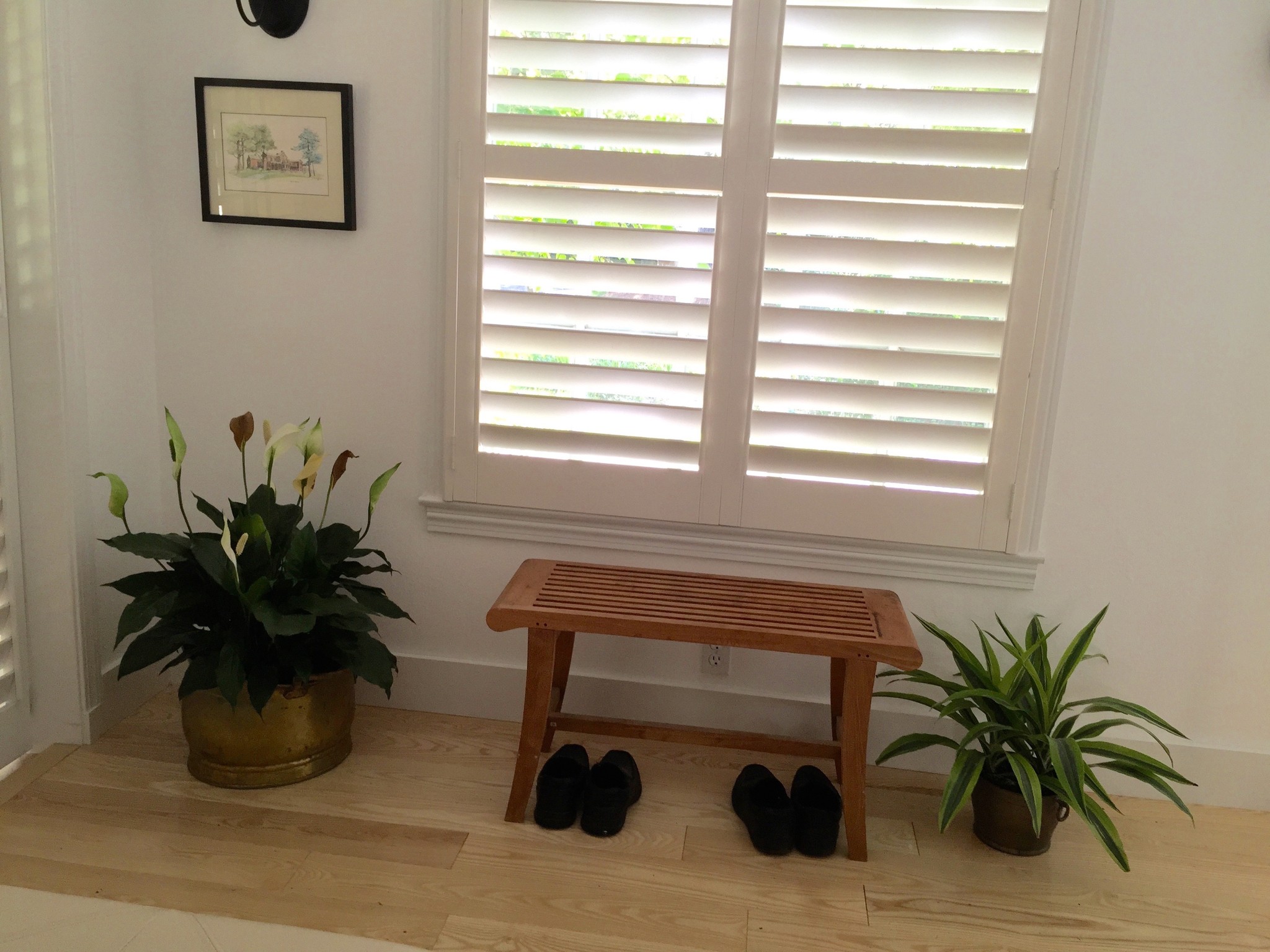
[867,605,1199,875]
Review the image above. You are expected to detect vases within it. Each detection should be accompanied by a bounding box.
[179,659,359,787]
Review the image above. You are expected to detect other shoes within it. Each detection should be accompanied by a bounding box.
[533,743,590,831]
[579,749,641,837]
[790,764,842,858]
[732,764,794,857]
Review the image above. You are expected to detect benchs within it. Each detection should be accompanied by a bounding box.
[486,559,925,864]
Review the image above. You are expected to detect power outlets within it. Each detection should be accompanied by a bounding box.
[700,644,730,677]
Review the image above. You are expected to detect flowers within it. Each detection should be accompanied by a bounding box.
[85,405,416,718]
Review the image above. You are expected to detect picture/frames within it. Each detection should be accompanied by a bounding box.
[193,76,358,233]
